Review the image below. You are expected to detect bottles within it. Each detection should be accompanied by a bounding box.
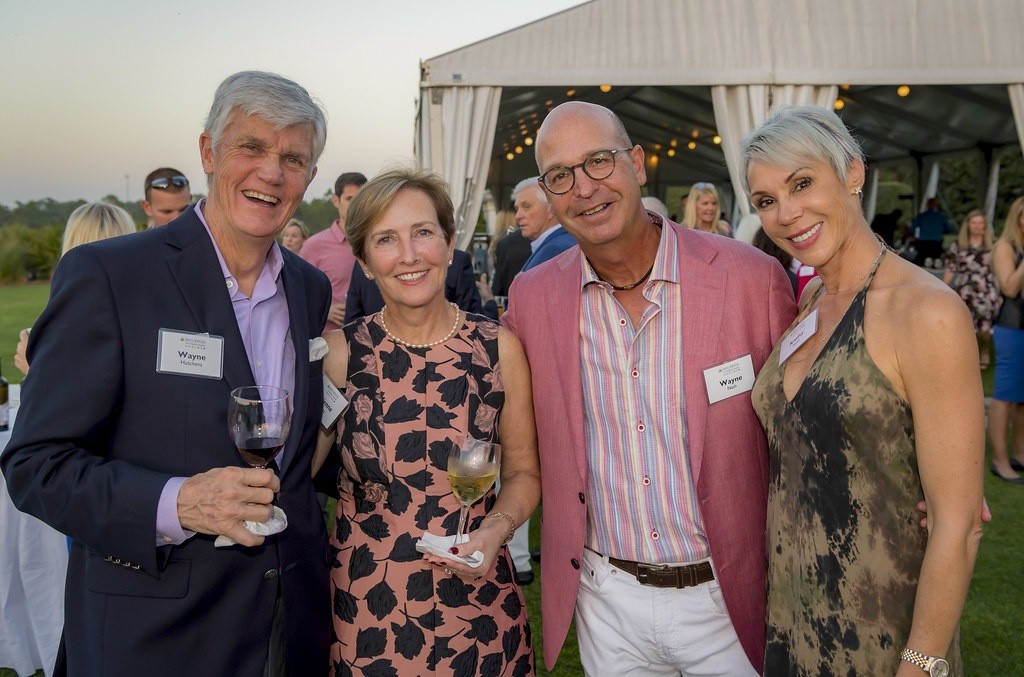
[0,357,9,432]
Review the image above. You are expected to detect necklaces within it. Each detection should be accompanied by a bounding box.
[586,213,663,291]
[380,302,461,349]
[788,243,884,362]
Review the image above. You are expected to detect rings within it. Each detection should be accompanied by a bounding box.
[444,567,455,576]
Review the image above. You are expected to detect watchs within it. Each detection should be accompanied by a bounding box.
[486,512,515,546]
[900,647,950,677]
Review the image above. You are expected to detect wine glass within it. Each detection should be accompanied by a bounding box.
[229,385,290,525]
[447,436,501,564]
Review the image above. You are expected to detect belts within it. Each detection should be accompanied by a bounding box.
[585,547,715,589]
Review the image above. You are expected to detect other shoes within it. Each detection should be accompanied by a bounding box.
[990,462,1024,485]
[1010,457,1024,472]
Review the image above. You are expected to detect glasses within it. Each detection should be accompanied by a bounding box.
[540,148,632,194]
[147,176,187,189]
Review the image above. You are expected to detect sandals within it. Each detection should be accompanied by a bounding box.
[980,363,989,372]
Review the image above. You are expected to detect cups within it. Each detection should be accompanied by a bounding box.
[495,297,508,319]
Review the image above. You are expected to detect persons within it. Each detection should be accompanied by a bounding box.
[488,176,581,297]
[869,208,903,250]
[736,102,987,677]
[679,179,734,236]
[0,73,332,677]
[140,168,192,231]
[943,207,1004,372]
[14,199,140,379]
[340,232,490,320]
[753,225,799,298]
[280,217,310,258]
[641,194,670,219]
[895,195,957,269]
[986,194,1024,484]
[298,170,369,334]
[315,171,539,677]
[495,99,993,676]
